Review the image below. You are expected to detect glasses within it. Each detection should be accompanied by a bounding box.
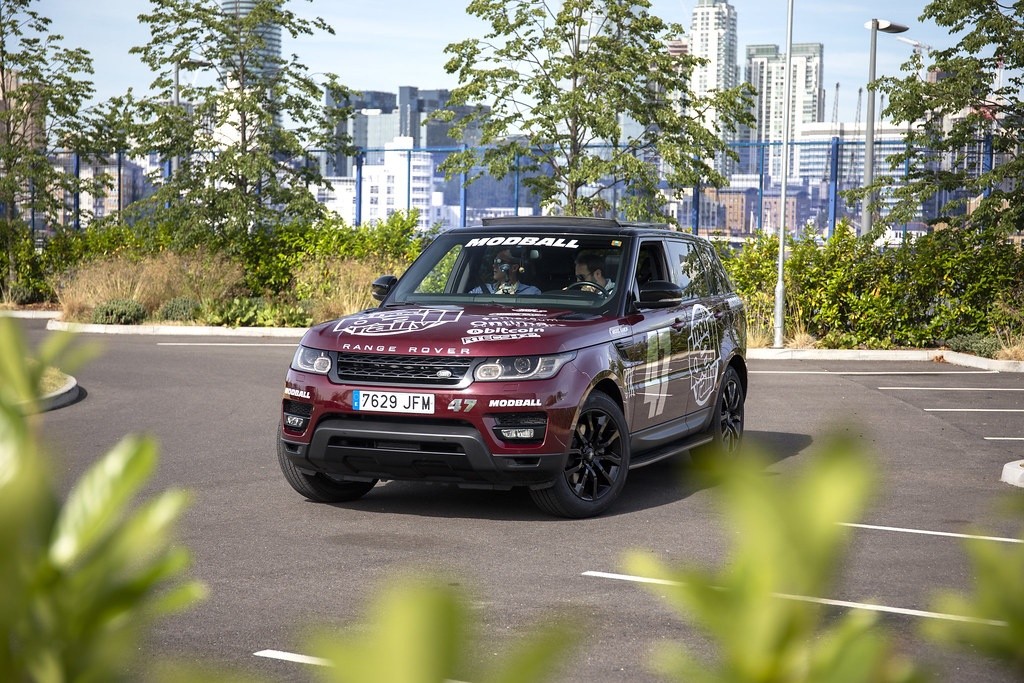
[576,269,602,281]
[494,258,512,266]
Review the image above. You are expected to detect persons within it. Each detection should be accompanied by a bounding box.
[468,248,542,295]
[573,250,615,298]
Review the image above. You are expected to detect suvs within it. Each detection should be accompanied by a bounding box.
[274,213,750,520]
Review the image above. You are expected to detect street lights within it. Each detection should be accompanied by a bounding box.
[860,18,913,262]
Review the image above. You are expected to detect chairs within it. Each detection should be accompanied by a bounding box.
[603,255,631,284]
